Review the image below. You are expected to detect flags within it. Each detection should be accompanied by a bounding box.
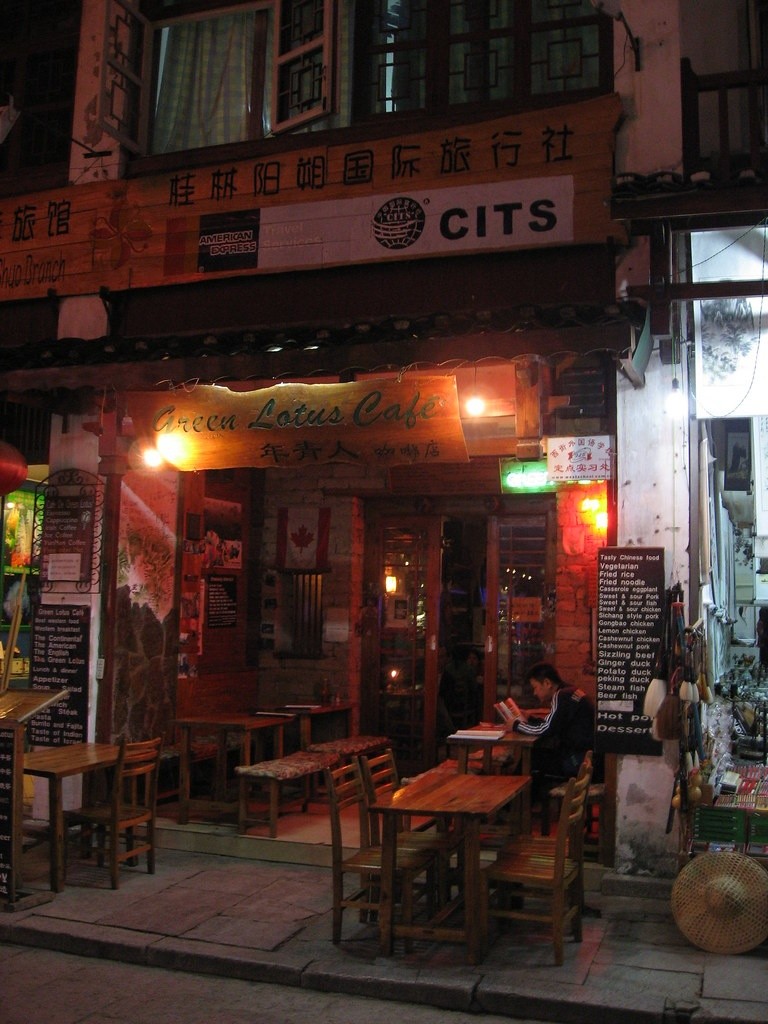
[274,505,331,574]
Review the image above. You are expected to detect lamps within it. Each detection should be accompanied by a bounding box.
[590,0,642,72]
[0,93,113,158]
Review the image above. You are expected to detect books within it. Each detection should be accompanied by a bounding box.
[447,722,508,740]
[493,698,526,723]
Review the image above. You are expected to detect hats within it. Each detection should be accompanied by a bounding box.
[672,851,768,955]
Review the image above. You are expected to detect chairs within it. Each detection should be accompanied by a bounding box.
[323,749,465,945]
[62,732,166,890]
[481,751,593,966]
[450,680,477,725]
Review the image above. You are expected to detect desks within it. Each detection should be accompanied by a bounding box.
[253,703,360,803]
[366,773,532,965]
[447,725,540,833]
[380,688,424,701]
[525,708,550,718]
[23,743,155,892]
[168,713,296,825]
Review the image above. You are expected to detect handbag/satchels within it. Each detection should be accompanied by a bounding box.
[655,667,685,740]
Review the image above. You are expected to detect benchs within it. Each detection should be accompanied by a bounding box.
[401,758,483,785]
[308,735,394,802]
[234,751,338,838]
[160,731,255,802]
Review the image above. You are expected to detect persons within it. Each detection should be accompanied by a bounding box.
[462,650,501,701]
[504,663,598,807]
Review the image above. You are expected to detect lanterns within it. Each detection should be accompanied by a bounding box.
[0,443,28,497]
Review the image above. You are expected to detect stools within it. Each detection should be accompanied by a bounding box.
[550,781,607,833]
